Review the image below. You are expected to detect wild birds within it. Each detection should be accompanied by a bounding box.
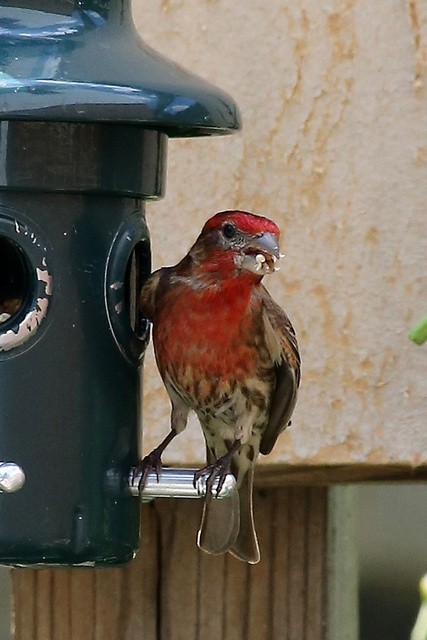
[129,210,303,565]
